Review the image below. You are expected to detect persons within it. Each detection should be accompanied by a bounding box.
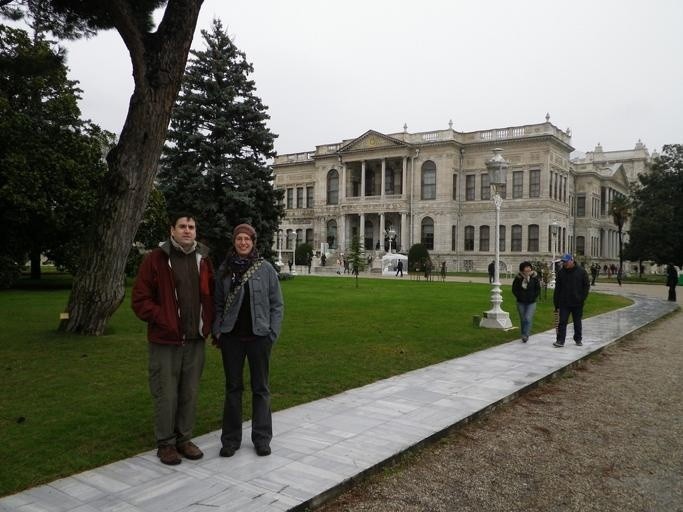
[551,253,590,346]
[285,236,448,282]
[487,260,495,283]
[209,222,285,457]
[132,212,207,464]
[666,261,678,302]
[590,261,627,286]
[511,261,541,343]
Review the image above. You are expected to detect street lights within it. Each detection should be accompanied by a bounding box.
[472,148,519,331]
[386,225,396,254]
[289,230,298,275]
[547,217,560,290]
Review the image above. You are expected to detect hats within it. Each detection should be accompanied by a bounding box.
[559,254,572,261]
[231,223,257,248]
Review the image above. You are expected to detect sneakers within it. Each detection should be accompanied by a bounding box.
[219,442,241,457]
[176,439,203,461]
[252,441,272,456]
[573,335,582,345]
[522,334,530,342]
[156,443,181,465]
[552,342,565,347]
